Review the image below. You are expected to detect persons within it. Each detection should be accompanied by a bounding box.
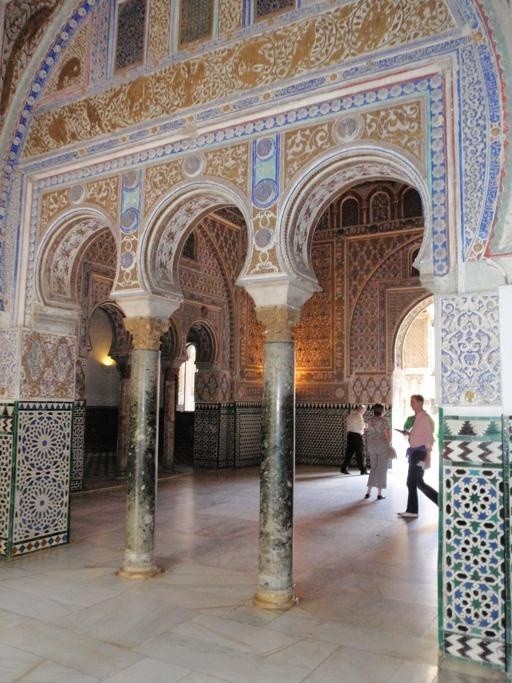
[396,393,438,518]
[363,401,392,500]
[338,403,370,476]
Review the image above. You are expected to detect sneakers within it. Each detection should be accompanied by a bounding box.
[365,494,368,498]
[397,512,418,518]
[378,496,386,499]
[361,472,369,475]
[340,469,350,474]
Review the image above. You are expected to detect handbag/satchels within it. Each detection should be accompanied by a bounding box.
[387,447,396,458]
